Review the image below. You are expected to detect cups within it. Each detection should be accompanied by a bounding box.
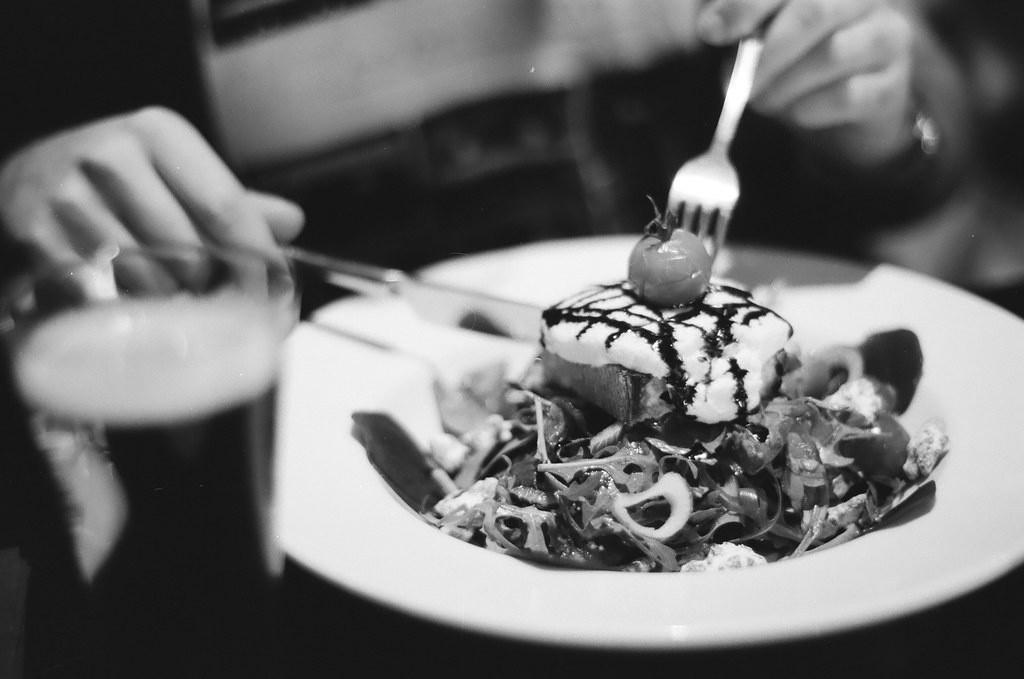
[0,241,292,679]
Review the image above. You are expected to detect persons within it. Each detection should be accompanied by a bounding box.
[0,0,1024,563]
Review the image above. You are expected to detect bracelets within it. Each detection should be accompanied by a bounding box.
[896,98,943,177]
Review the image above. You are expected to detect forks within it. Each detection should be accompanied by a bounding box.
[664,36,765,249]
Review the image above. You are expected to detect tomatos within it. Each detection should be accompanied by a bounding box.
[628,199,713,305]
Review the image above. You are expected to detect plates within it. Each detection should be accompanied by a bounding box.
[275,232,1024,650]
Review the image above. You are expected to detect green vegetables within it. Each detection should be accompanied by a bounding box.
[416,370,949,572]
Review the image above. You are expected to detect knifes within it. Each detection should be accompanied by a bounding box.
[279,247,550,343]
